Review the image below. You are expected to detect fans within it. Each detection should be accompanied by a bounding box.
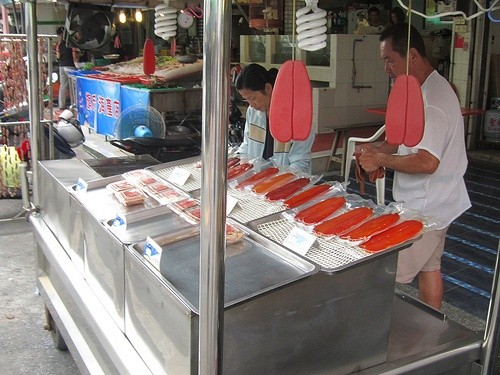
[114,105,166,139]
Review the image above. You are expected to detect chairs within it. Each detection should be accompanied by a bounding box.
[345,126,394,204]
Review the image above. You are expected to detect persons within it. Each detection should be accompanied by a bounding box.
[357,21,474,311]
[369,7,385,26]
[109,23,119,54]
[389,6,406,24]
[56,27,86,63]
[0,135,24,197]
[56,41,80,110]
[233,63,316,180]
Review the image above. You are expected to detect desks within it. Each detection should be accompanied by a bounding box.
[67,70,203,137]
[368,105,483,143]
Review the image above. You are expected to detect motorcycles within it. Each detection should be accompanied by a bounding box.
[105,69,246,165]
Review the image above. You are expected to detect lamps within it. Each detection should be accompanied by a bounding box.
[295,0,328,51]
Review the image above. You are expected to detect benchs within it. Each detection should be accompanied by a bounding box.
[327,121,383,176]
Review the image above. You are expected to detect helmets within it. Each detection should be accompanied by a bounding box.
[68,5,112,50]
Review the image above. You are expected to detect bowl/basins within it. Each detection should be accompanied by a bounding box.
[167,126,191,135]
